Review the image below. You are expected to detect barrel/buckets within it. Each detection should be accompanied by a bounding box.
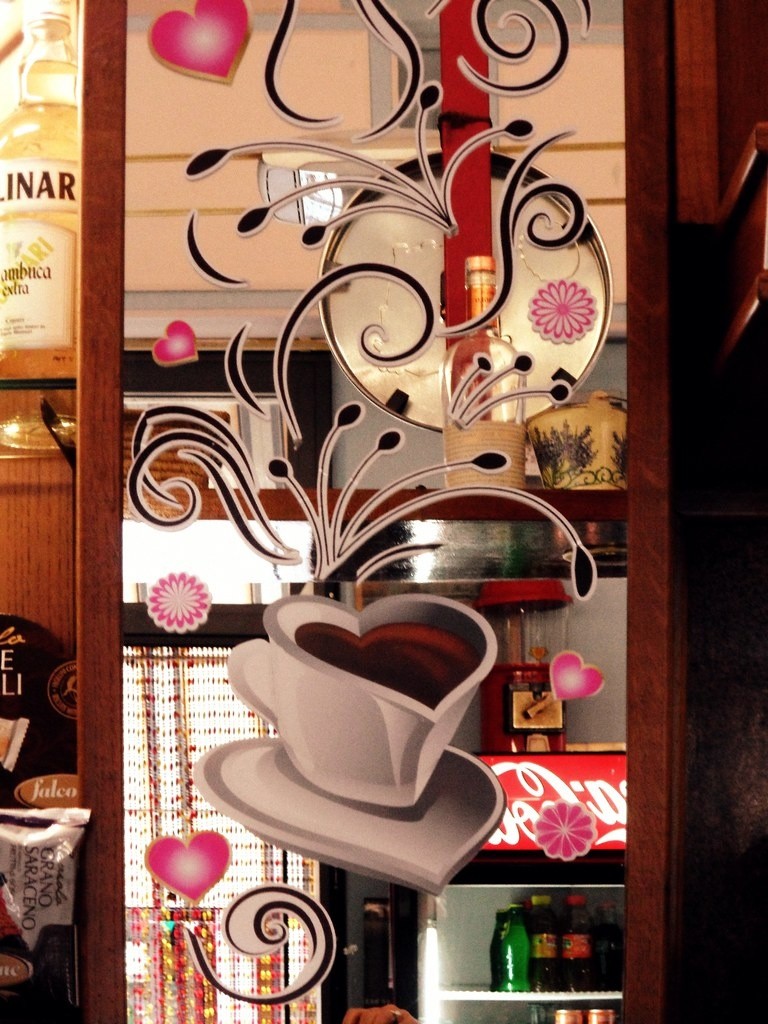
[524,393,628,489]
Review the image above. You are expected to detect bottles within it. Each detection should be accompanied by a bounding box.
[492,892,622,995]
[441,255,528,498]
